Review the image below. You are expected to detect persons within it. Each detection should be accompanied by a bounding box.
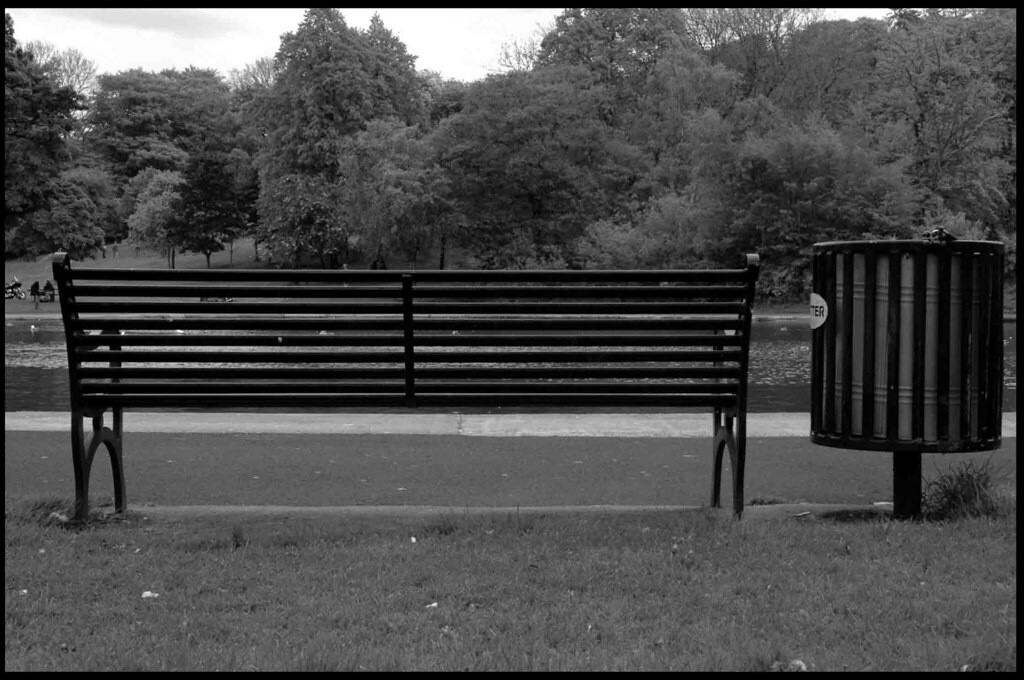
[31,281,39,296]
[112,241,118,258]
[44,280,54,302]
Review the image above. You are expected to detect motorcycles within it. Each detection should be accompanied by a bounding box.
[5,276,27,300]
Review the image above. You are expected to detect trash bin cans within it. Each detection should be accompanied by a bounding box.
[808,235,1007,451]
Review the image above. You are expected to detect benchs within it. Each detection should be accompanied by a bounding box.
[51,250,760,521]
